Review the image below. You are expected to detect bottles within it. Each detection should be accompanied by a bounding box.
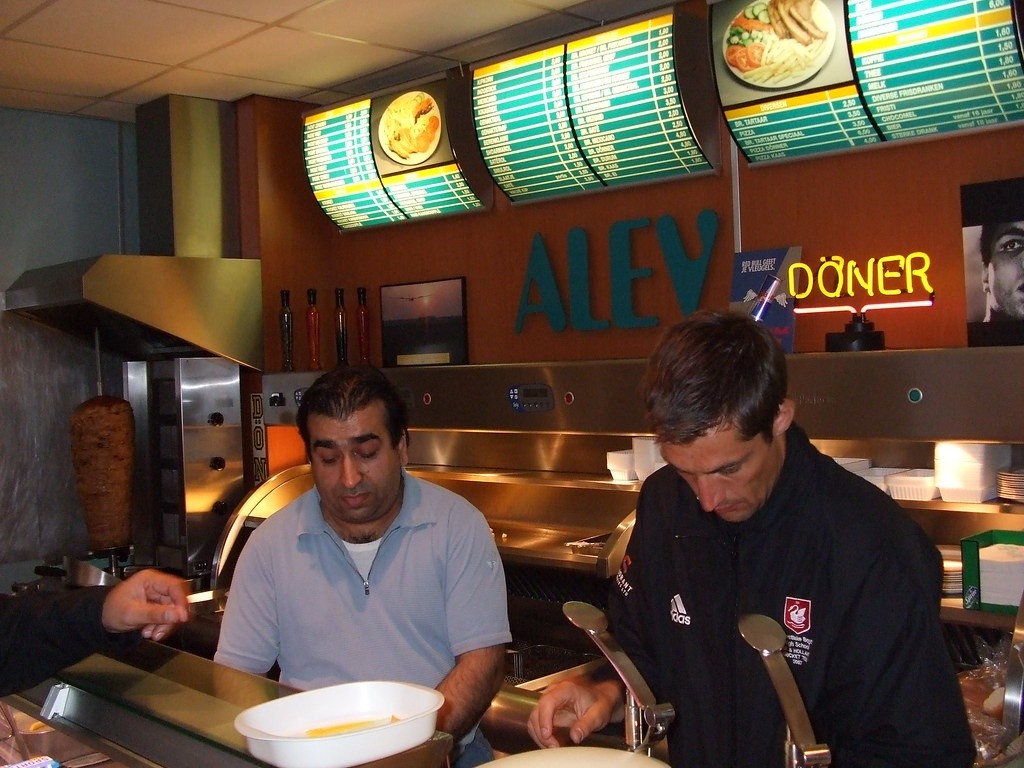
[357,287,370,361]
[335,288,347,363]
[306,288,321,371]
[280,290,293,370]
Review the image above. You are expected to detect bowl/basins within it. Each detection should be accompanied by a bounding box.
[233,680,445,768]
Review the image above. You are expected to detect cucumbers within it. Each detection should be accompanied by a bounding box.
[744,3,770,23]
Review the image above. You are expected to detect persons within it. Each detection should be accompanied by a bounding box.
[1,568,189,698]
[967,221,1024,347]
[526,308,978,768]
[214,363,513,768]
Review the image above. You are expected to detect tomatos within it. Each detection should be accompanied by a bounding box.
[726,43,763,71]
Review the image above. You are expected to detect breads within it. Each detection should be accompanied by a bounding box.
[767,0,827,45]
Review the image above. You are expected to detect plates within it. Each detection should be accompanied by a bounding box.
[996,467,1024,503]
[936,544,962,594]
